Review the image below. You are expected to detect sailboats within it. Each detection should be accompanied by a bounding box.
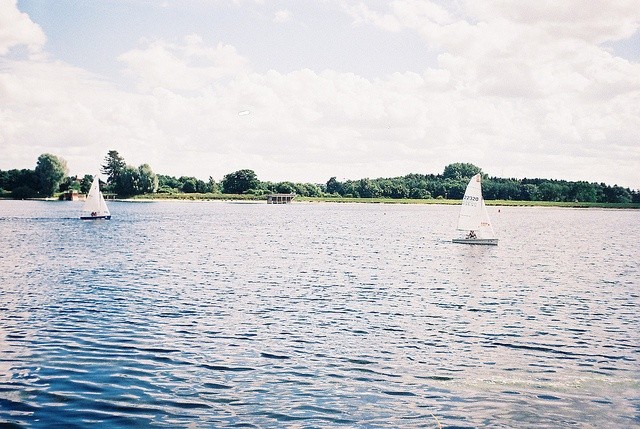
[452,173,499,246]
[80,173,111,221]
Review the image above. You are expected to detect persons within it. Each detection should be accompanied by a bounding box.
[468,230,477,239]
